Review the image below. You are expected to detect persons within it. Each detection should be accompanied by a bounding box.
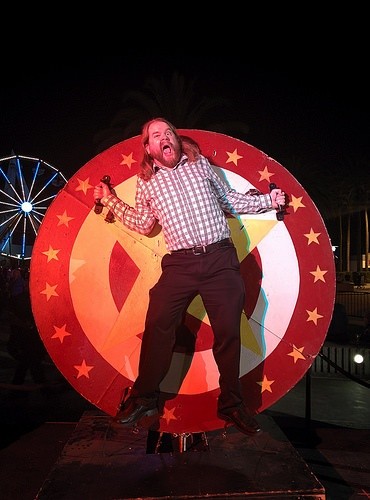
[93,117,286,436]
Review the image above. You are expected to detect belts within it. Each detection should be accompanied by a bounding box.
[171,238,234,255]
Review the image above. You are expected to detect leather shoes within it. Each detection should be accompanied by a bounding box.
[218,403,262,436]
[113,401,160,427]
